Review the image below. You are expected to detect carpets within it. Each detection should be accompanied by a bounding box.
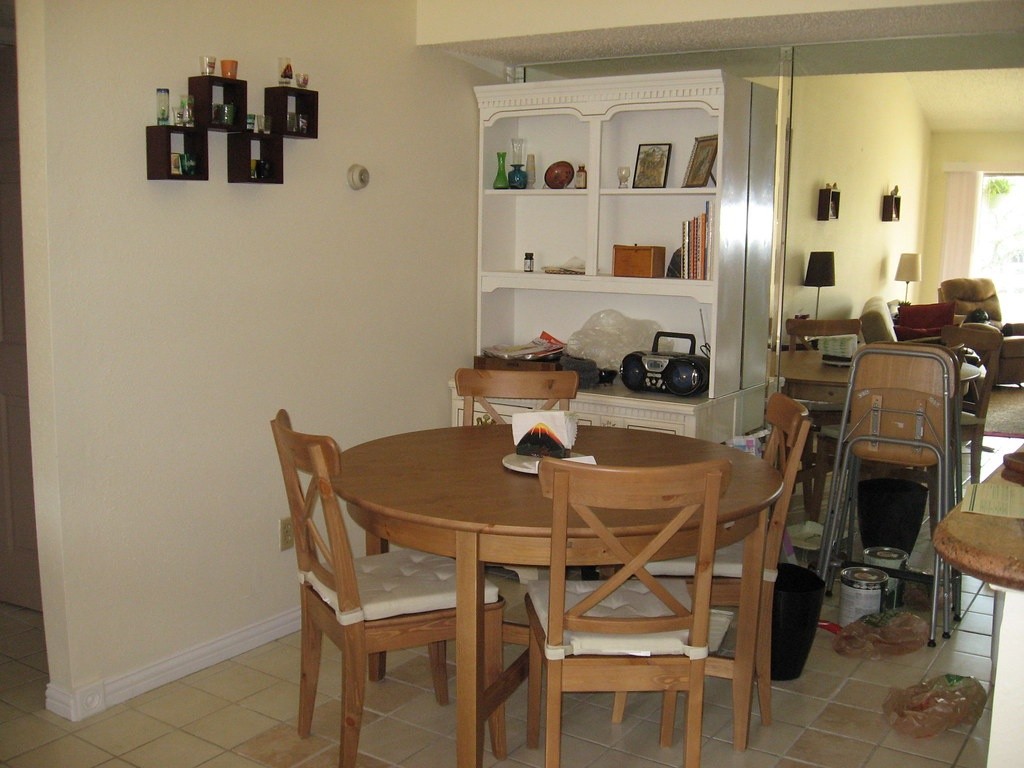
[982,386,1024,438]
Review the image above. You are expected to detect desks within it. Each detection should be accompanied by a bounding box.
[779,347,981,585]
[768,349,851,522]
[330,424,783,768]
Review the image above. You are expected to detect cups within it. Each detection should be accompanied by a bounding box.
[173,107,183,125]
[171,154,179,174]
[251,159,256,178]
[296,73,308,89]
[297,114,307,133]
[180,96,194,125]
[200,55,216,75]
[278,57,291,85]
[180,154,196,173]
[257,115,271,134]
[247,114,255,132]
[257,160,269,179]
[287,112,295,131]
[221,60,237,79]
[215,104,233,123]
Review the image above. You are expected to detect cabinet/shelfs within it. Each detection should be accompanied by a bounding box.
[145,74,320,186]
[451,69,739,443]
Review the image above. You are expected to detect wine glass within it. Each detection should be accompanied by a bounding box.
[617,167,629,188]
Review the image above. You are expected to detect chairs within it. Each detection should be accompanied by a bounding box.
[610,391,813,753]
[785,317,864,524]
[523,453,736,768]
[456,369,616,764]
[812,343,960,651]
[270,410,508,768]
[940,323,1004,489]
[827,341,964,649]
[859,294,952,345]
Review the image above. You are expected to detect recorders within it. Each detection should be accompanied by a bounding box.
[619,330,710,396]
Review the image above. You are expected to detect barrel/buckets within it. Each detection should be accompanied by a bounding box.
[838,566,889,626]
[863,547,908,608]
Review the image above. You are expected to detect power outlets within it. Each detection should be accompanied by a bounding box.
[278,516,294,552]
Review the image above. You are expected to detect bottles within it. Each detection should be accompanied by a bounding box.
[493,138,536,189]
[574,164,586,188]
[156,88,169,125]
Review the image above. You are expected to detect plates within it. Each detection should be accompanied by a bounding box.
[545,161,574,188]
[502,453,587,474]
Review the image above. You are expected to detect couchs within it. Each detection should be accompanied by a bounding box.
[937,276,1024,385]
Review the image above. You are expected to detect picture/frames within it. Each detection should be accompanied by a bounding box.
[681,135,718,188]
[632,143,672,188]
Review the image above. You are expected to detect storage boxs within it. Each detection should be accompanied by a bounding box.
[612,243,666,277]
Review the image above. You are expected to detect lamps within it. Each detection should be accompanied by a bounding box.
[803,251,837,320]
[894,253,922,303]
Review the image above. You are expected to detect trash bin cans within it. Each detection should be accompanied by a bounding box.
[773,561,825,681]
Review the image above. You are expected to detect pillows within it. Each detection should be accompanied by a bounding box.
[893,324,943,341]
[898,300,956,327]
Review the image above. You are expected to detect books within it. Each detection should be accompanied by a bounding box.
[682,200,713,281]
